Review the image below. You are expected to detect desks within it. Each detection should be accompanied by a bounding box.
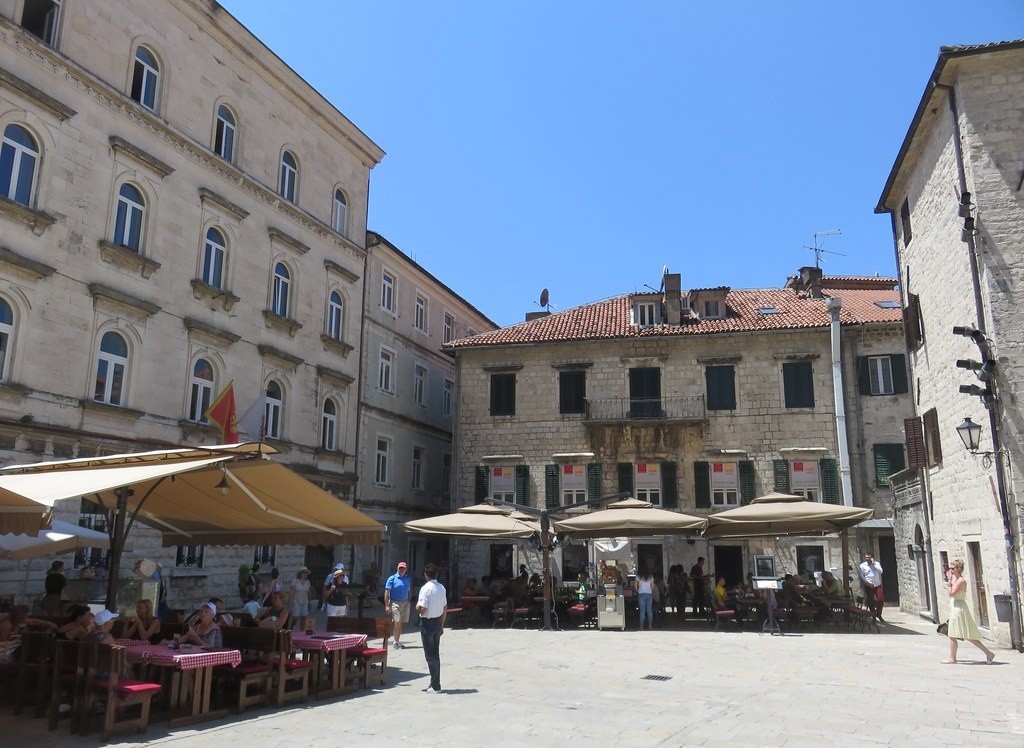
[808,591,854,626]
[461,594,492,622]
[115,639,243,727]
[292,629,368,700]
[735,597,764,628]
[535,594,567,625]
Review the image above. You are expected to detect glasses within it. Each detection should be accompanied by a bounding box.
[948,568,956,570]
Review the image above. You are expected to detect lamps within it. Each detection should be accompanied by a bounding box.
[956,417,1011,468]
[961,216,979,242]
[951,325,996,410]
[958,192,977,218]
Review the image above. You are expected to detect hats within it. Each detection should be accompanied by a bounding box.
[296,566,311,579]
[200,602,217,616]
[332,569,344,579]
[334,563,345,570]
[397,561,408,568]
[93,609,120,626]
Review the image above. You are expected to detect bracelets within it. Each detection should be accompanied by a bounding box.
[948,578,952,580]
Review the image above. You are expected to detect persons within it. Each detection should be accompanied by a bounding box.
[575,573,587,604]
[289,567,311,631]
[460,564,557,627]
[416,563,447,694]
[600,563,623,585]
[385,562,411,650]
[715,553,846,626]
[652,557,705,622]
[0,591,290,713]
[245,564,283,607]
[634,564,655,629]
[76,564,107,600]
[859,552,884,622]
[941,556,995,665]
[40,561,76,616]
[323,563,349,617]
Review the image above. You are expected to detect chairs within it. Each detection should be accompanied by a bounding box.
[47,638,92,734]
[705,589,883,629]
[17,627,68,716]
[79,640,162,742]
[446,583,597,629]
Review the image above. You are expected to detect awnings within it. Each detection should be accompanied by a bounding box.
[0,519,134,562]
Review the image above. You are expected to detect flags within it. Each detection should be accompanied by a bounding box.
[204,379,238,445]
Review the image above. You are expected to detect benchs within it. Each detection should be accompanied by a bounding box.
[29,614,390,712]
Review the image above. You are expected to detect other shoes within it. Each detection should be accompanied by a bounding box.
[421,684,441,694]
[647,626,653,630]
[398,643,406,649]
[873,617,876,621]
[878,617,884,622]
[941,656,957,665]
[639,626,643,630]
[392,643,398,650]
[985,653,995,665]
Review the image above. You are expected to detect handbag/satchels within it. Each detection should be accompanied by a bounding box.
[937,618,965,641]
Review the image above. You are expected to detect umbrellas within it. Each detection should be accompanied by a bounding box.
[396,497,558,629]
[708,491,875,622]
[0,441,385,612]
[547,496,712,628]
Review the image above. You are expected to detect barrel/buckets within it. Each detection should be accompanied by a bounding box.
[994,595,1013,622]
